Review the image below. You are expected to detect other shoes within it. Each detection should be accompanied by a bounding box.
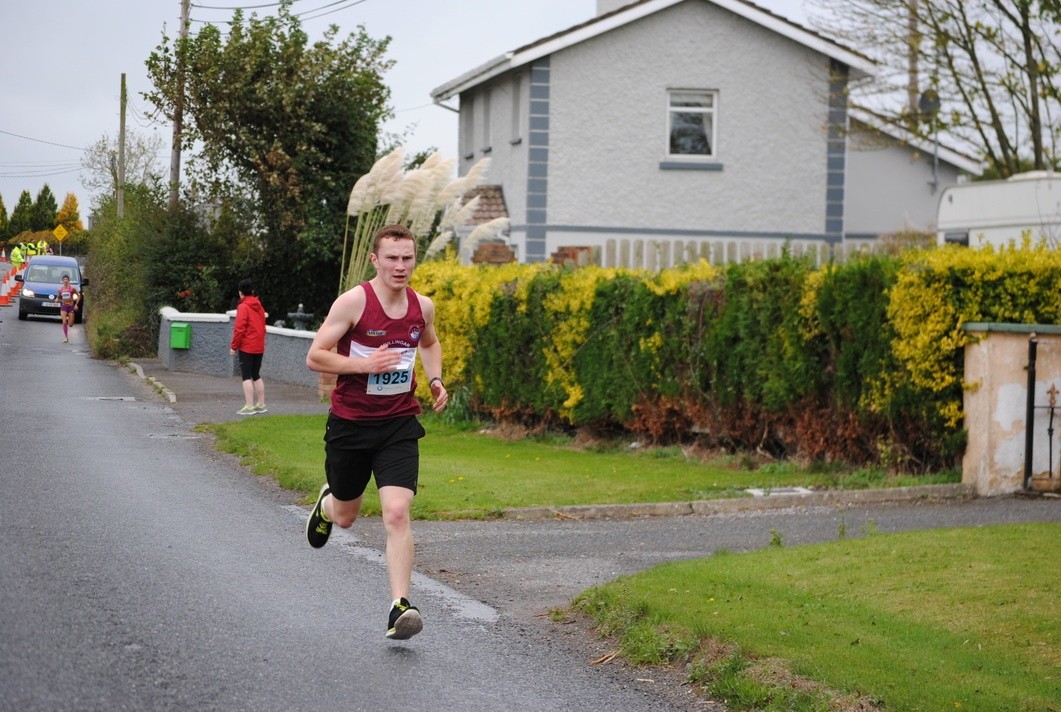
[64,339,68,342]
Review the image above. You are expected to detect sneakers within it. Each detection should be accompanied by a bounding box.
[236,405,256,414]
[385,597,422,639]
[306,483,333,549]
[254,402,268,413]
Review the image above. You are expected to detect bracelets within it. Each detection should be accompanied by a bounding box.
[429,377,444,387]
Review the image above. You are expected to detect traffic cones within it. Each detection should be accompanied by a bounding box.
[0,276,13,307]
[5,272,16,303]
[10,262,29,297]
[0,247,7,262]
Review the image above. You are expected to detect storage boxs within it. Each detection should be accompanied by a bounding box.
[170,321,191,350]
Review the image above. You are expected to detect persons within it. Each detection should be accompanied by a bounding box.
[30,270,45,282]
[10,238,54,271]
[55,276,80,343]
[230,278,268,415]
[306,224,448,639]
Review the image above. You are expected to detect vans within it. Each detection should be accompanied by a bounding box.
[15,255,90,324]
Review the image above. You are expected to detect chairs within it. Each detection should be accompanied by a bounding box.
[60,271,70,278]
